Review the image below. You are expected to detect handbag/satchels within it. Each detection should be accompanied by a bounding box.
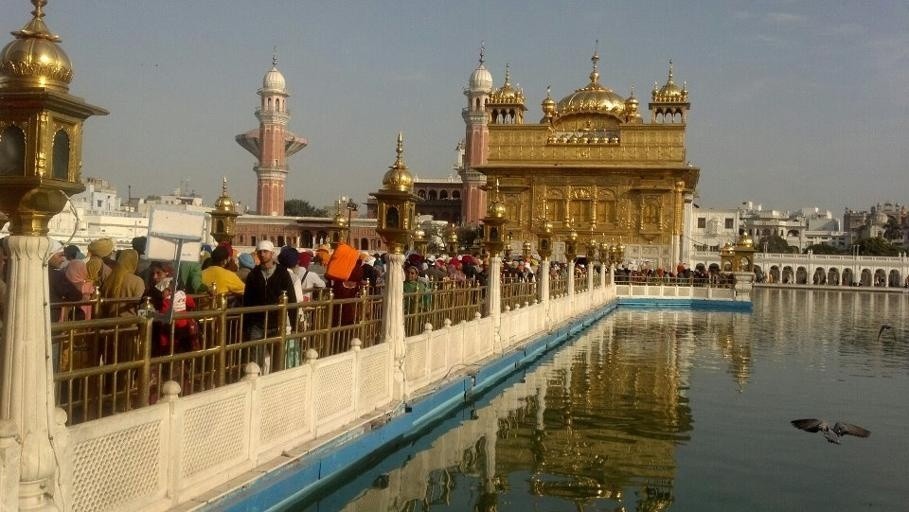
[334,281,358,298]
[324,244,360,283]
[187,318,203,350]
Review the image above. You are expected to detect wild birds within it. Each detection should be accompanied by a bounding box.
[877,324,897,343]
[789,418,872,445]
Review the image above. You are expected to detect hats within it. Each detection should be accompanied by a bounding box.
[155,277,175,292]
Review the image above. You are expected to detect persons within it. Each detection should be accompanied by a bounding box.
[614,261,729,288]
[49,236,588,413]
